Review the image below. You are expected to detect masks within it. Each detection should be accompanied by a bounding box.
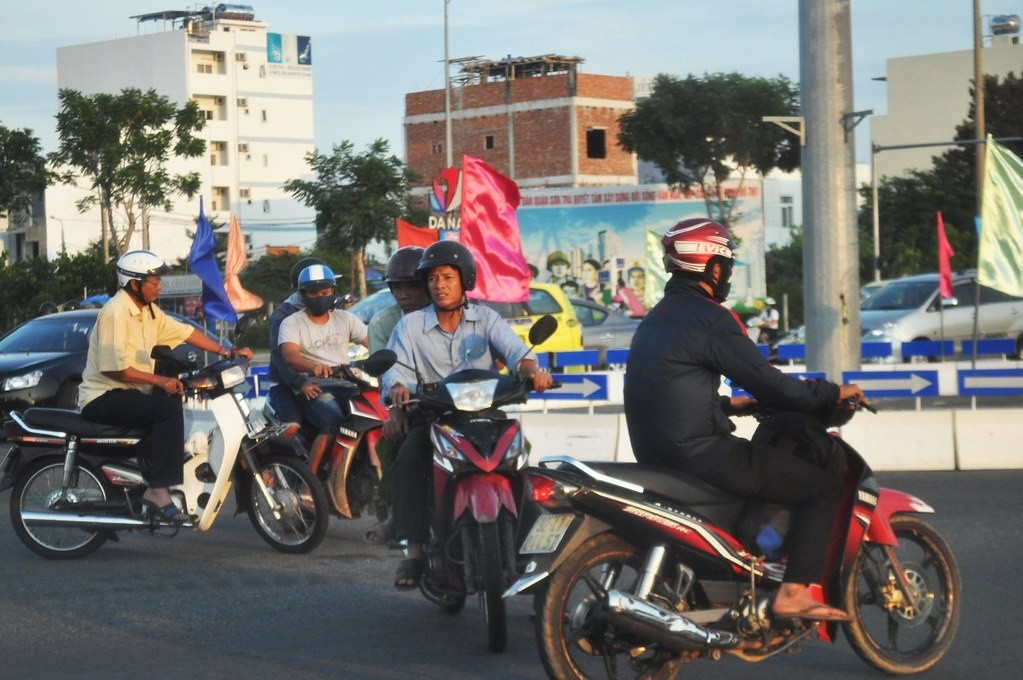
[708,270,731,302]
[305,291,336,315]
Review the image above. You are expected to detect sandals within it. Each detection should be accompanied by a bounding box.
[394,558,426,591]
[363,522,394,545]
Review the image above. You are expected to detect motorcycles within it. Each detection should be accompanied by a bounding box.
[0,309,331,561]
[499,389,964,679]
[364,313,564,654]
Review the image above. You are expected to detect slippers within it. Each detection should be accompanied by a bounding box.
[299,489,315,511]
[770,603,853,621]
[143,498,188,522]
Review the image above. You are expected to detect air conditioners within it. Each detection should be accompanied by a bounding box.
[239,189,250,197]
[214,53,222,60]
[217,143,224,150]
[237,99,247,106]
[218,187,226,195]
[216,97,224,104]
[236,53,246,61]
[238,144,248,151]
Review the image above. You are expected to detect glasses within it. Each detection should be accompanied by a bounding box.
[146,279,162,287]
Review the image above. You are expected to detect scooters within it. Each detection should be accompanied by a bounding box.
[263,363,390,523]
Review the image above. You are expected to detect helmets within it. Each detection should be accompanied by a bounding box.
[63,301,81,311]
[417,240,475,297]
[39,303,58,316]
[662,217,737,276]
[291,258,325,289]
[297,264,336,296]
[385,246,425,294]
[116,250,171,287]
[765,297,776,305]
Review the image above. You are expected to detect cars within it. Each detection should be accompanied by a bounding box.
[0,307,236,428]
[769,273,1023,365]
[343,282,644,374]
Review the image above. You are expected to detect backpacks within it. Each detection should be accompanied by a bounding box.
[735,411,847,561]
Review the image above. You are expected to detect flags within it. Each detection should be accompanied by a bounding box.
[397,219,439,248]
[188,214,238,323]
[223,219,263,311]
[978,137,1023,297]
[938,210,953,298]
[462,156,531,302]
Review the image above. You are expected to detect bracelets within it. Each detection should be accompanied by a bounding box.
[224,350,229,359]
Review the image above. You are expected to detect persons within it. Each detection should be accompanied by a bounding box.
[270,258,369,512]
[39,300,103,315]
[368,241,553,591]
[623,218,868,622]
[759,299,779,344]
[72,251,254,521]
